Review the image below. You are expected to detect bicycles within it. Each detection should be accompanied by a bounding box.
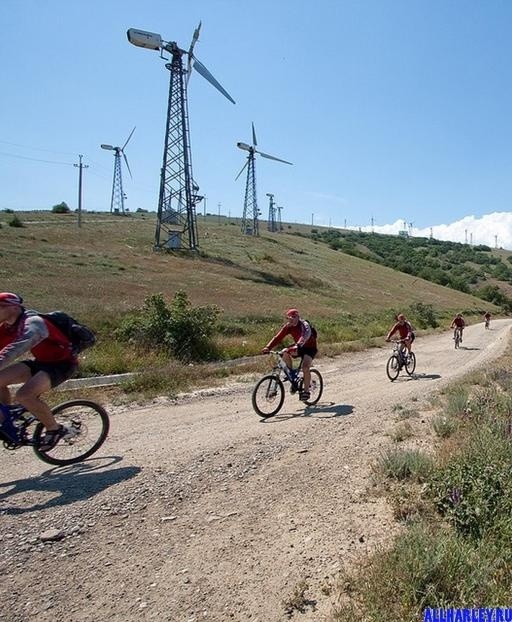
[0,393,110,466]
[450,326,464,350]
[485,319,489,329]
[252,351,323,418]
[386,340,416,380]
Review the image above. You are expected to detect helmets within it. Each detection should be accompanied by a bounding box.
[397,314,406,319]
[0,292,23,306]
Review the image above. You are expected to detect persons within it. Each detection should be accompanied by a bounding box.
[261,309,318,401]
[483,311,492,326]
[386,314,415,371]
[451,313,465,343]
[0,292,80,453]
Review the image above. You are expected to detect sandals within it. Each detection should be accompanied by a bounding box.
[36,424,69,452]
[301,391,310,401]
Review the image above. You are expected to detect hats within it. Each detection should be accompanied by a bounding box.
[287,309,300,316]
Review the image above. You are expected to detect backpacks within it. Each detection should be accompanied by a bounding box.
[304,319,317,338]
[22,310,97,355]
